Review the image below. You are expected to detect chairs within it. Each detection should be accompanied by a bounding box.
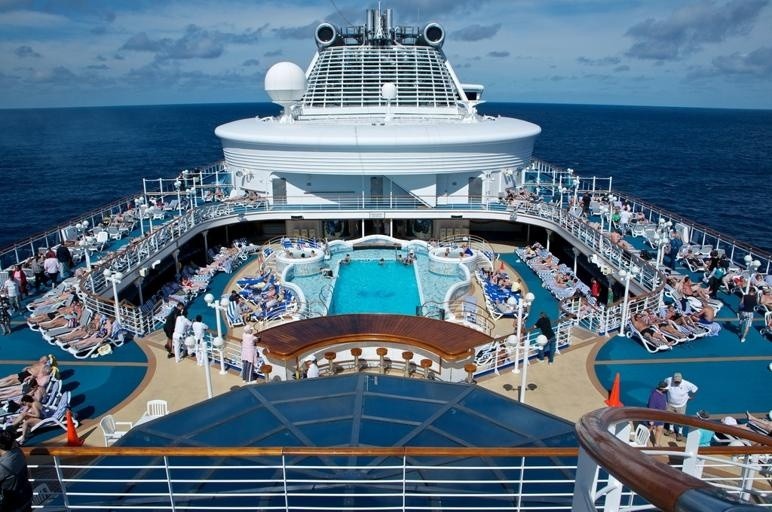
[474,268,528,323]
[141,260,298,324]
[1,187,140,448]
[686,417,771,471]
[629,424,651,448]
[263,236,319,259]
[131,185,266,260]
[143,399,169,416]
[475,337,523,370]
[625,297,720,356]
[497,185,771,338]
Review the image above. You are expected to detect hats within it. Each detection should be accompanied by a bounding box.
[674,373,682,383]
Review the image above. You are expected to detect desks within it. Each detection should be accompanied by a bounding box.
[134,415,159,426]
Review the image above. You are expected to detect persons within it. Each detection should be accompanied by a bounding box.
[664,372,698,441]
[442,185,772,366]
[395,253,418,265]
[746,409,772,433]
[320,268,339,280]
[343,254,352,264]
[1,429,34,512]
[378,258,384,265]
[1,169,318,446]
[647,382,671,446]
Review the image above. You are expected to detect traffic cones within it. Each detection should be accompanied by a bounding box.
[604,371,625,409]
[506,332,548,403]
[62,409,84,448]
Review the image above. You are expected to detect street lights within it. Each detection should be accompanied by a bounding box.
[571,178,581,211]
[134,195,144,235]
[181,169,189,188]
[183,334,225,399]
[652,233,668,280]
[608,193,618,232]
[741,252,761,295]
[617,265,641,338]
[75,221,89,239]
[598,203,608,243]
[185,187,196,226]
[566,167,574,186]
[201,292,230,375]
[506,293,535,374]
[103,267,123,325]
[258,176,271,210]
[145,205,156,246]
[659,216,672,264]
[173,180,182,216]
[558,185,567,222]
[192,176,201,208]
[79,236,95,272]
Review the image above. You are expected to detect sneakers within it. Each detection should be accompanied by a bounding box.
[677,433,683,441]
[664,430,673,436]
